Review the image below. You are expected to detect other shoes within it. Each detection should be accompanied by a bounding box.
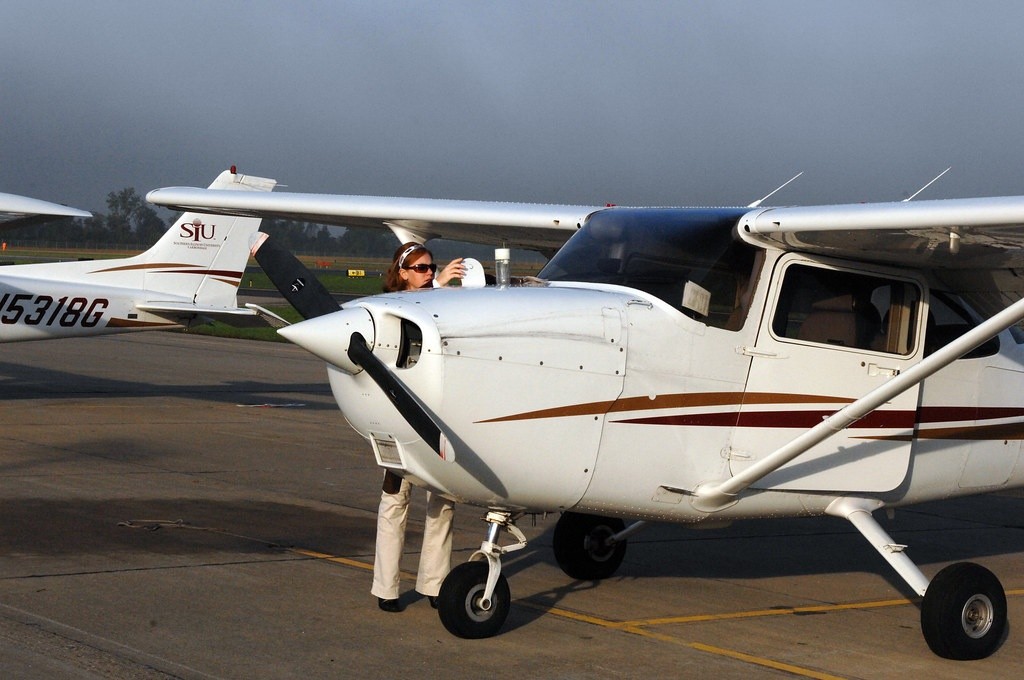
[379,597,400,612]
[428,595,438,608]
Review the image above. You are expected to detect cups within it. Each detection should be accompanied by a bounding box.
[494,248,512,288]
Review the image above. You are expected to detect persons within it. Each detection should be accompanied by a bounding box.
[369,242,470,617]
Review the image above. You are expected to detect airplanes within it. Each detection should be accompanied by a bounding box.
[1,169,277,341]
[144,186,1023,661]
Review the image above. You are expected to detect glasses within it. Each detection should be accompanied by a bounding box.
[402,264,437,273]
